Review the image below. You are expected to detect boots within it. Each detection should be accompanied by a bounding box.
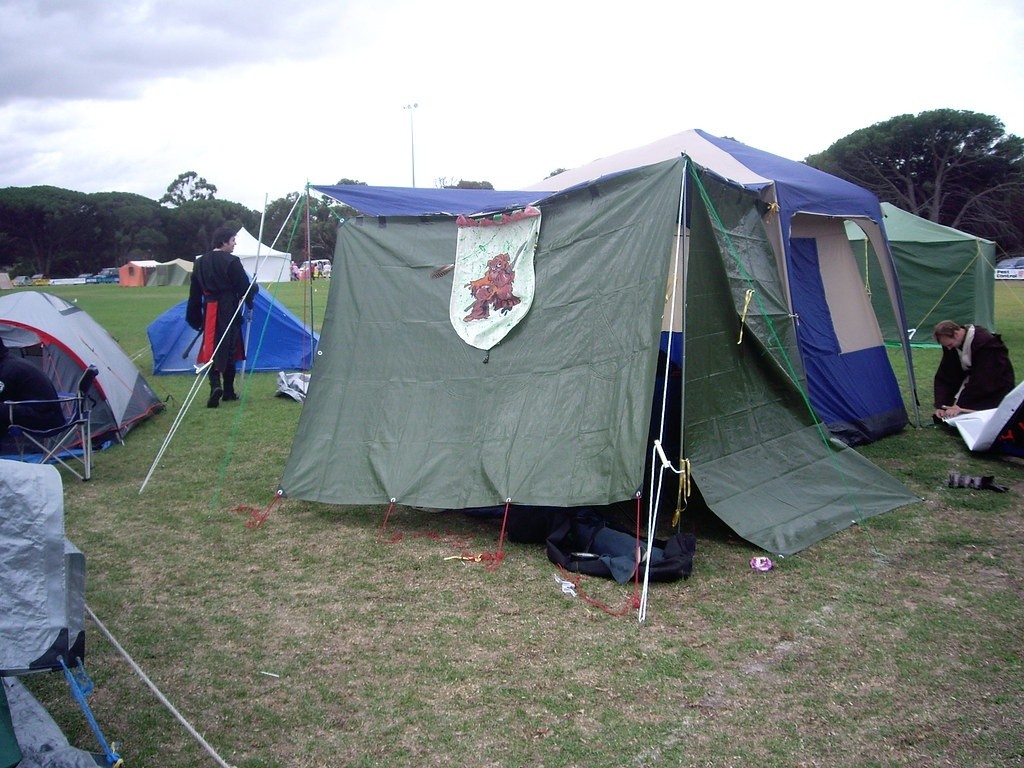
[222,371,240,401]
[207,369,223,408]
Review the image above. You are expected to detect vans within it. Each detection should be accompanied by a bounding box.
[298,259,331,272]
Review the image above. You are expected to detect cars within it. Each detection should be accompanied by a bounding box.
[996,257,1024,269]
[32,273,52,279]
[12,275,30,285]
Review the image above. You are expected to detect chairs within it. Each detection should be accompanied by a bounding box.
[4,365,100,483]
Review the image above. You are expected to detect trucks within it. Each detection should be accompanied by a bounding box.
[94,268,120,279]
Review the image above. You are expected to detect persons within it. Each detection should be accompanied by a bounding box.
[933,319,1016,419]
[185,227,260,408]
[0,337,67,455]
[290,259,331,283]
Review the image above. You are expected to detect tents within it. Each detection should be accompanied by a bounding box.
[119,258,194,287]
[0,291,167,678]
[271,124,995,561]
[147,281,320,375]
[194,225,291,283]
[0,273,48,289]
